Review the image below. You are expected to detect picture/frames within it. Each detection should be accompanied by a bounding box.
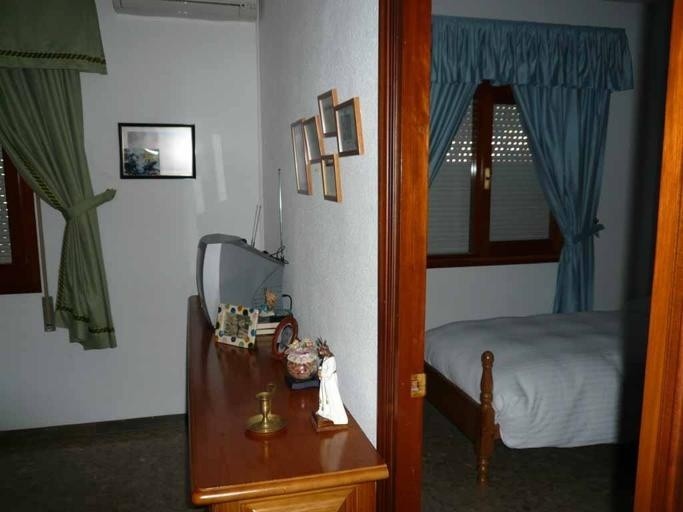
[321,153,341,202]
[317,88,340,137]
[271,315,298,361]
[334,96,364,157]
[289,117,312,196]
[303,114,325,164]
[215,301,259,349]
[118,122,196,180]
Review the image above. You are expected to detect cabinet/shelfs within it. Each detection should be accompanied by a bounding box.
[186,294,390,511]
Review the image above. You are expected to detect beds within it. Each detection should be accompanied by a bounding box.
[424,311,626,487]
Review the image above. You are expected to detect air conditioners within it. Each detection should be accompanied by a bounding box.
[113,0,257,21]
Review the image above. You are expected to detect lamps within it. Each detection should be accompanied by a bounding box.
[265,291,292,322]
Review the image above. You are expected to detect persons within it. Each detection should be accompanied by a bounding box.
[274,324,294,354]
[124,147,159,177]
[314,343,349,426]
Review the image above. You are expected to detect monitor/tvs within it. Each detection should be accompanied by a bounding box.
[196,234,284,336]
